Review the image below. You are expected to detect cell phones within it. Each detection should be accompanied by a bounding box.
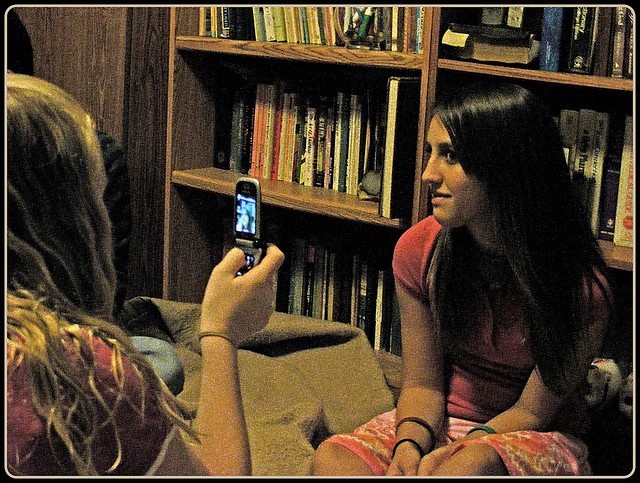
[232,176,268,278]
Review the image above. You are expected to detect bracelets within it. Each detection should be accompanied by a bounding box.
[395,416,436,454]
[392,439,424,458]
[466,425,496,435]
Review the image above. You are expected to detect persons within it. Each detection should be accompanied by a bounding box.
[1,11,130,334]
[237,207,255,234]
[4,72,287,475]
[309,81,615,479]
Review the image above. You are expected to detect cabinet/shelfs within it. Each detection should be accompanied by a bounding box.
[163,7,433,404]
[418,7,634,424]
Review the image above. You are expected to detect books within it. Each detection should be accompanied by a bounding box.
[480,7,506,26]
[546,107,636,249]
[442,23,538,66]
[539,7,634,79]
[261,221,408,357]
[506,6,525,28]
[218,224,235,256]
[198,5,426,55]
[213,63,419,219]
